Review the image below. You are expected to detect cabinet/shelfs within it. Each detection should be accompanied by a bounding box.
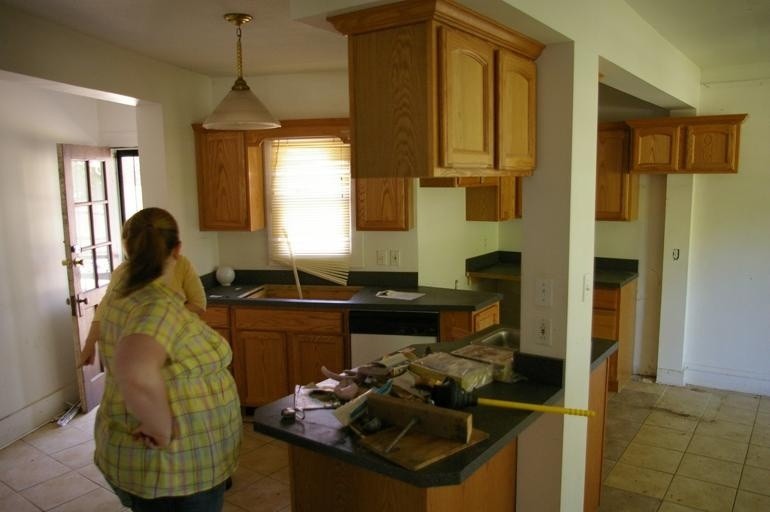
[629,124,738,173]
[232,307,344,408]
[441,304,500,342]
[590,279,636,393]
[348,24,538,175]
[596,128,639,220]
[197,129,265,233]
[357,173,416,231]
[465,175,515,221]
[194,305,230,351]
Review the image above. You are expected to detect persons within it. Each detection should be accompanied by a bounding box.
[94,207,242,511]
[73,216,235,492]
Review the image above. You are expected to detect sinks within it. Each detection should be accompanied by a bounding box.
[237,281,364,302]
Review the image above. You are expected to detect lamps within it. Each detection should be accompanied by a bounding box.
[201,12,283,134]
